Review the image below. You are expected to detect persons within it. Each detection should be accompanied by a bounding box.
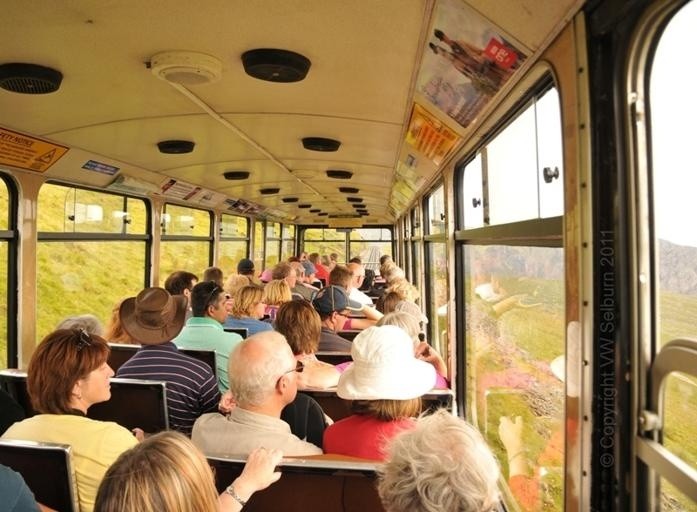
[302,259,318,291]
[102,299,137,345]
[299,252,317,275]
[237,259,255,277]
[288,263,312,295]
[224,275,250,296]
[272,263,303,295]
[264,280,293,320]
[328,265,370,312]
[375,255,419,311]
[174,280,243,393]
[499,353,566,512]
[311,286,363,352]
[222,286,273,337]
[117,287,222,439]
[0,328,144,512]
[309,253,329,286]
[164,270,198,323]
[0,464,58,511]
[93,430,283,512]
[351,258,375,289]
[375,408,501,512]
[323,325,437,461]
[203,268,224,287]
[345,263,374,306]
[191,331,323,460]
[321,256,332,272]
[335,311,448,390]
[273,299,341,393]
[396,302,428,329]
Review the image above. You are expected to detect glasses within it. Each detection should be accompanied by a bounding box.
[202,279,219,310]
[76,327,92,352]
[419,331,426,342]
[284,360,305,374]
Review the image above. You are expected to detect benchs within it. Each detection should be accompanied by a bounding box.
[301,390,370,424]
[223,328,249,338]
[199,459,391,512]
[0,371,169,445]
[104,343,215,388]
[3,443,80,512]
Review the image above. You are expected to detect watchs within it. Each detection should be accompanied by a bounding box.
[225,485,246,507]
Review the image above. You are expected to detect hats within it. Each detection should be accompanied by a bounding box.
[428,42,439,55]
[119,286,189,345]
[300,260,318,276]
[237,259,256,272]
[335,324,437,401]
[392,298,429,324]
[434,29,444,42]
[312,285,364,312]
